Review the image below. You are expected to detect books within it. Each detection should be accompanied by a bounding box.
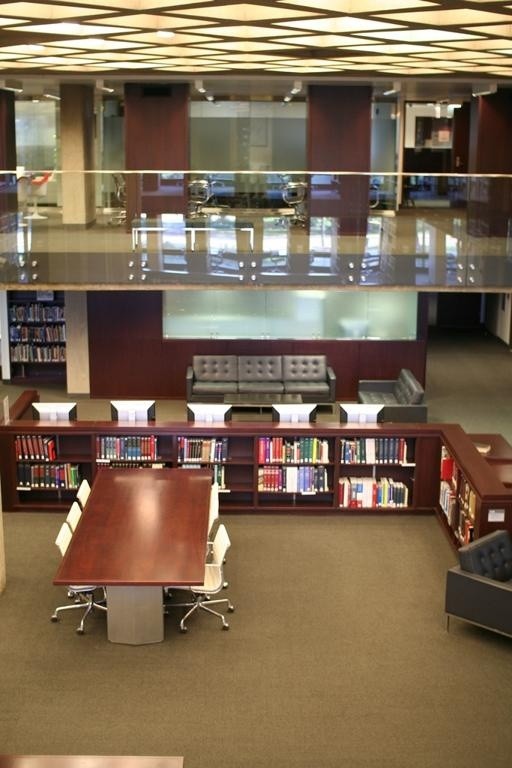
[97,462,165,469]
[454,506,474,552]
[8,303,65,322]
[16,463,79,490]
[178,464,225,490]
[341,438,407,463]
[339,477,408,508]
[457,472,476,523]
[177,437,227,462]
[258,466,329,492]
[439,481,452,527]
[258,436,329,463]
[10,344,67,362]
[10,324,67,342]
[97,435,157,462]
[440,444,458,492]
[15,435,56,461]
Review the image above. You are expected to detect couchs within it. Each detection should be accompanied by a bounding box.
[186,355,336,402]
[358,369,428,422]
[445,530,512,639]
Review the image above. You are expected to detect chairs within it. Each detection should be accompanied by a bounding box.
[76,479,92,508]
[163,524,235,633]
[108,172,129,225]
[188,175,216,219]
[23,170,51,220]
[278,171,308,228]
[368,177,385,209]
[65,501,82,531]
[205,480,227,565]
[50,523,107,635]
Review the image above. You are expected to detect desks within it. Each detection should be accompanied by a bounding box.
[53,469,214,645]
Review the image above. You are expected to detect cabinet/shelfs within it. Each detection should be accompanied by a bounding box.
[7,290,67,377]
[434,426,511,549]
[0,420,459,515]
